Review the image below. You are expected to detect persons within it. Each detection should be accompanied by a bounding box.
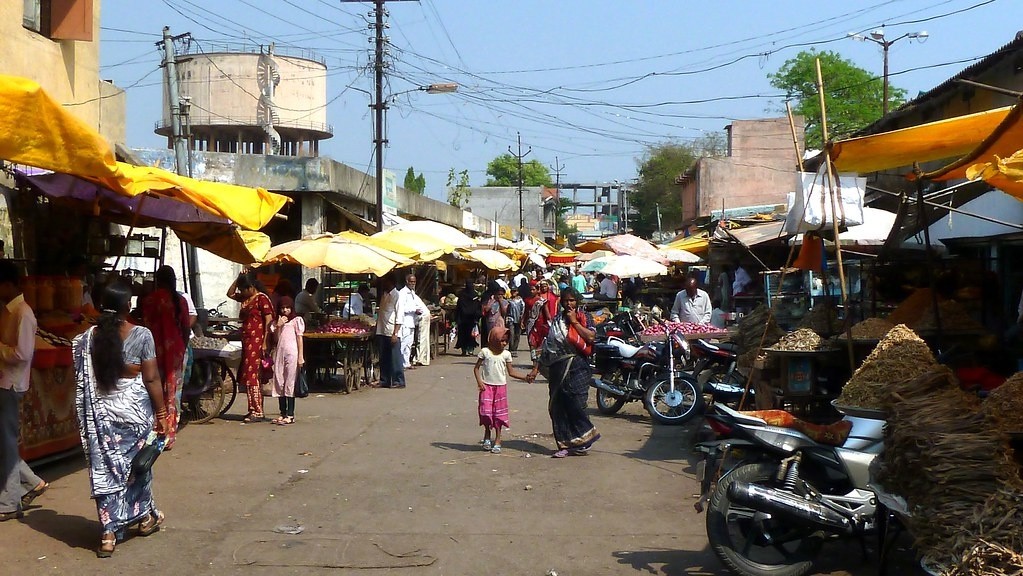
[268,296,305,425]
[669,277,712,323]
[0,257,49,522]
[473,327,534,455]
[342,283,370,320]
[227,269,275,422]
[372,272,431,389]
[454,259,753,371]
[141,265,198,451]
[528,286,602,458]
[294,278,329,325]
[72,275,169,558]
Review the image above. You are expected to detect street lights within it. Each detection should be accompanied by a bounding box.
[376,84,459,232]
[604,180,628,234]
[846,31,930,116]
[519,195,554,240]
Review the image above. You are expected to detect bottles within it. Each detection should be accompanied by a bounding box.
[20,274,82,311]
[87,234,159,296]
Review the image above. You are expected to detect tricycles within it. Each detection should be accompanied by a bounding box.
[180,356,238,424]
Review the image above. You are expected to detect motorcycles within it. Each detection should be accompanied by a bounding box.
[694,394,916,575]
[207,299,231,331]
[589,309,702,425]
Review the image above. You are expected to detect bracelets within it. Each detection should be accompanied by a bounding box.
[155,406,169,419]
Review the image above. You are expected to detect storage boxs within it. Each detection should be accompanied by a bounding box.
[596,344,619,372]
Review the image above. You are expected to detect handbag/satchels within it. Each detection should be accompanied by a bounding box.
[295,366,310,398]
[567,311,593,357]
[260,351,275,370]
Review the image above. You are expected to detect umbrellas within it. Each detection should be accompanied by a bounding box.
[243,219,520,320]
[573,248,705,278]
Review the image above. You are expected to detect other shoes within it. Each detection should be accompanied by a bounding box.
[244,415,263,423]
[372,384,389,388]
[271,415,286,424]
[552,448,570,459]
[483,440,493,450]
[467,347,474,355]
[390,384,405,389]
[461,348,466,357]
[491,445,502,454]
[276,416,296,424]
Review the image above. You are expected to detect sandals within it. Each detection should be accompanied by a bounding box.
[135,510,165,537]
[96,538,116,557]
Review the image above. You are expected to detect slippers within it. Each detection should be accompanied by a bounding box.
[21,482,51,511]
[0,509,23,523]
